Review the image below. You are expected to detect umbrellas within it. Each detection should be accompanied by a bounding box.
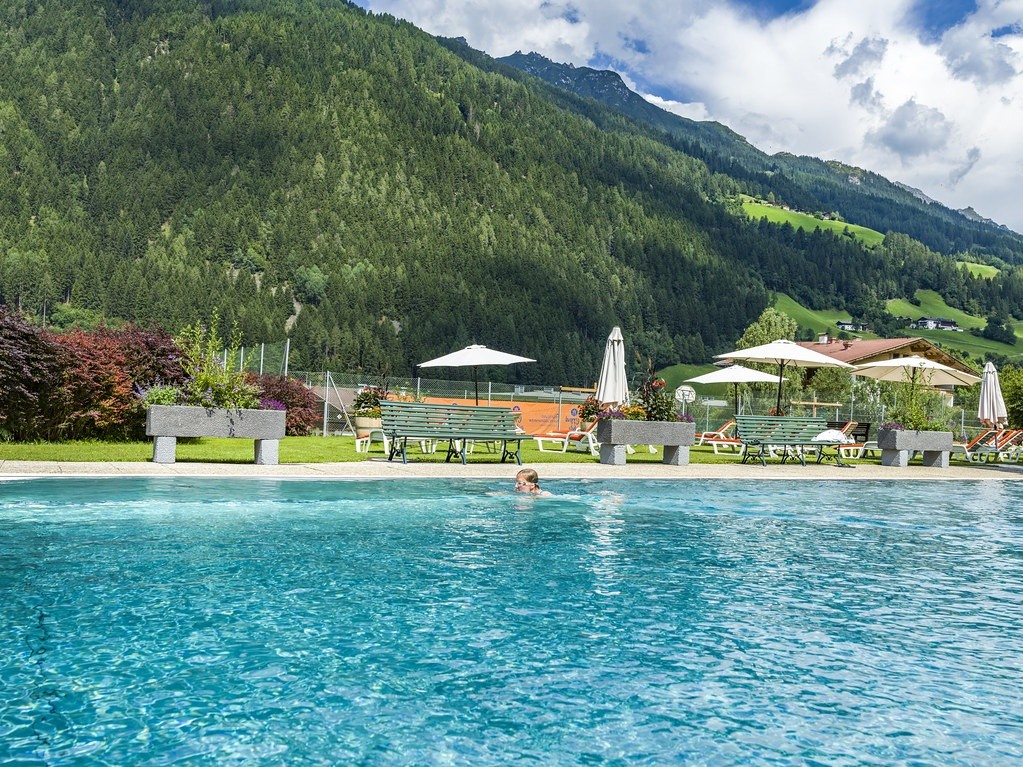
[682,364,790,439]
[711,339,859,418]
[850,354,987,414]
[415,344,538,407]
[594,326,630,413]
[977,362,1010,451]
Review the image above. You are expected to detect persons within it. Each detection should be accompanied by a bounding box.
[486,468,551,496]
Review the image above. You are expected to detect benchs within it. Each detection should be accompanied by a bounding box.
[826,422,871,444]
[732,413,853,469]
[378,399,534,466]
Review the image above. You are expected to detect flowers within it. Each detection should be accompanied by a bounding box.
[878,407,953,432]
[596,376,694,424]
[351,386,389,419]
[141,358,287,422]
[577,394,600,423]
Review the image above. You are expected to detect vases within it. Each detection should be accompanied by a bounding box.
[352,416,383,438]
[597,418,697,447]
[877,429,955,451]
[579,422,598,436]
[144,405,287,440]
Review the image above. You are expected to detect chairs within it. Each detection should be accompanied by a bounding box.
[694,421,884,460]
[531,412,605,458]
[948,427,1023,465]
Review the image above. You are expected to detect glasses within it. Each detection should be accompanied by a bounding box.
[516,481,534,486]
